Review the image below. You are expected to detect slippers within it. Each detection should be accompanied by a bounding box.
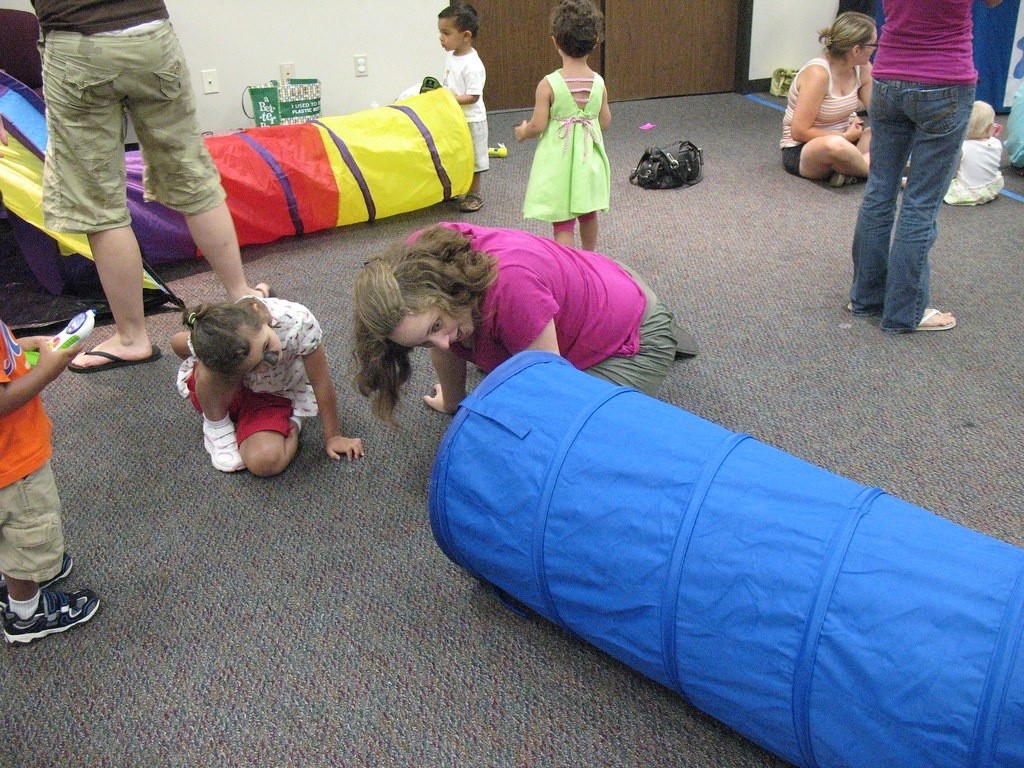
[254,285,276,298]
[67,345,161,373]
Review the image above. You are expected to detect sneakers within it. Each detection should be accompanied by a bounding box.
[0,551,73,614]
[1,588,100,647]
[460,192,484,211]
[203,419,247,472]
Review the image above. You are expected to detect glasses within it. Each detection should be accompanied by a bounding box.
[864,40,879,48]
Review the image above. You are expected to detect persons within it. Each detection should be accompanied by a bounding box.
[0,320,102,647]
[515,0,611,252]
[170,296,365,478]
[1003,84,1024,176]
[348,222,700,428]
[780,10,910,189]
[437,4,490,212]
[30,0,275,374]
[848,0,1001,335]
[942,101,1006,206]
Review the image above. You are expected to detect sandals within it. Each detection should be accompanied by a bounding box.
[915,309,956,330]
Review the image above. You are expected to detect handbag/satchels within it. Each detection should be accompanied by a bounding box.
[629,140,705,190]
[770,66,801,98]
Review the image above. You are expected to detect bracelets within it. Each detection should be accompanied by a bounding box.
[843,132,847,139]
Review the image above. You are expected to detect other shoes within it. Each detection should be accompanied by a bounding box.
[675,323,699,355]
[830,172,857,187]
